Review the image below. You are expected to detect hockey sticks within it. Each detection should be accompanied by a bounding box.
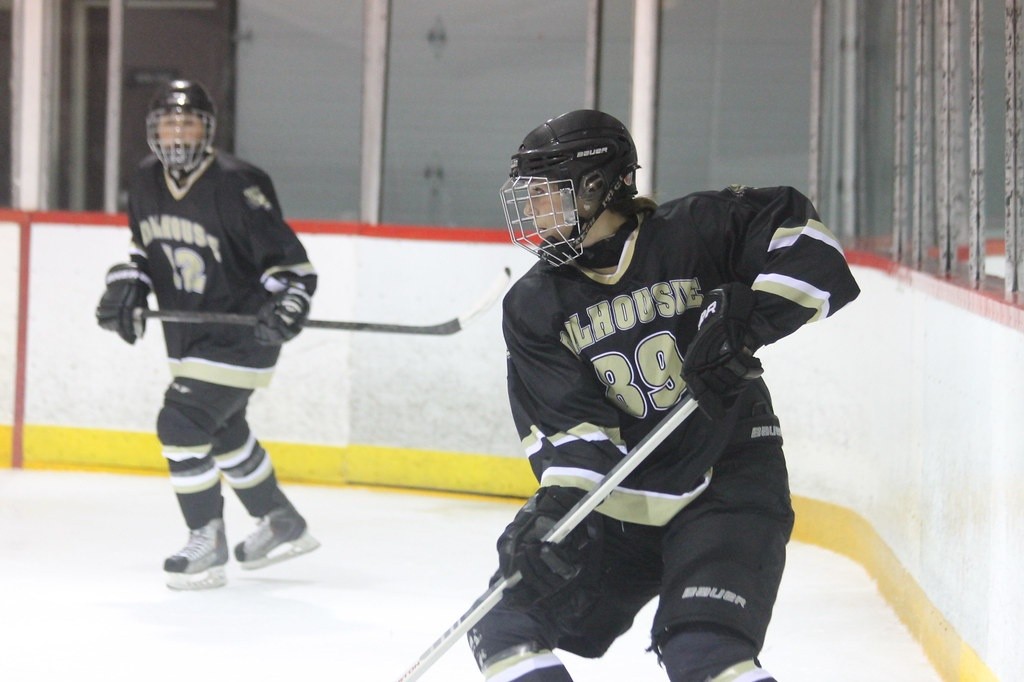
[396,344,754,682]
[131,304,462,342]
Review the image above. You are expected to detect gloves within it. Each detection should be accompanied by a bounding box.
[681,280,764,425]
[496,484,602,608]
[252,285,310,345]
[96,263,155,343]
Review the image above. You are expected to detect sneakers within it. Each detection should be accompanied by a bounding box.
[163,518,229,574]
[236,508,308,562]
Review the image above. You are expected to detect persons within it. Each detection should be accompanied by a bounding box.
[96,80,320,592]
[465,109,860,682]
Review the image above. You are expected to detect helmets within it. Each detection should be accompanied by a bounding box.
[146,78,216,171]
[500,109,637,269]
